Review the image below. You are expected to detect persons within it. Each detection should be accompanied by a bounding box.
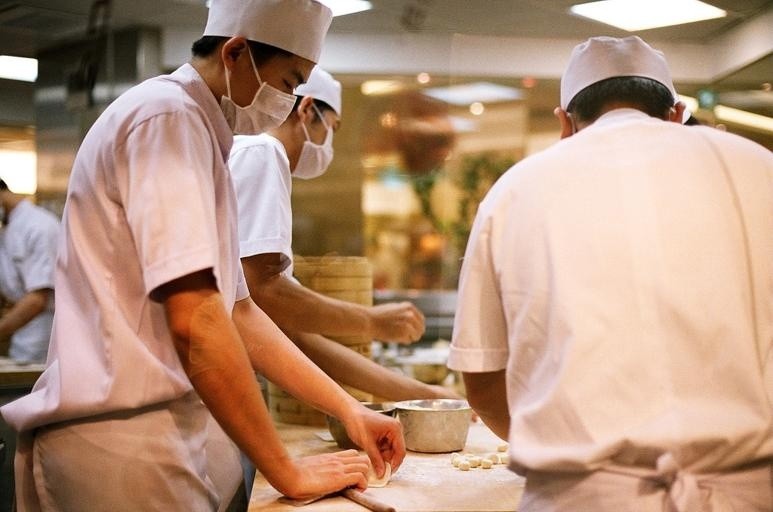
[445,35,773,512]
[0,179,63,367]
[227,64,479,426]
[0,1,407,512]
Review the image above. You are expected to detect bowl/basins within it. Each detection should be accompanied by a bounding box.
[323,400,395,450]
[392,398,472,453]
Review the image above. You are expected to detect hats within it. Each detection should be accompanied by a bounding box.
[559,34,677,112]
[203,0,333,65]
[293,65,343,118]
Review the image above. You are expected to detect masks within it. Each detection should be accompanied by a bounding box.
[219,43,298,136]
[291,104,334,180]
